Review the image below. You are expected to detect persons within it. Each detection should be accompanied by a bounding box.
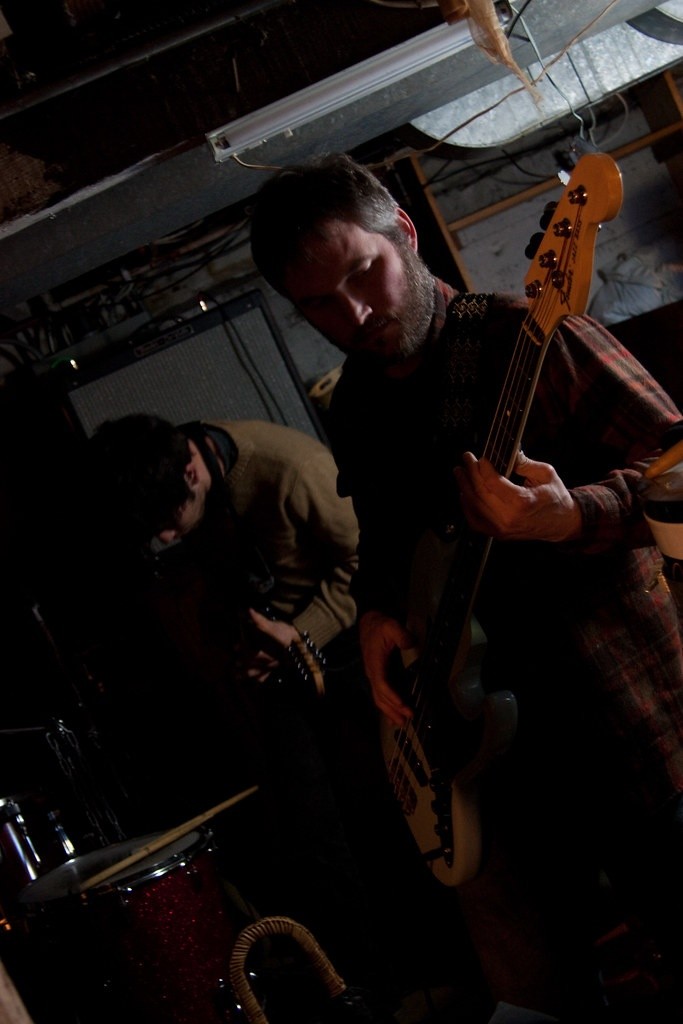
[250,148,683,983]
[79,411,359,687]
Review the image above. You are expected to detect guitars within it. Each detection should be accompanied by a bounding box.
[276,633,329,697]
[374,147,627,889]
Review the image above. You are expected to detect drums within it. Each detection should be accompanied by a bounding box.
[12,822,269,1024]
[0,793,50,933]
